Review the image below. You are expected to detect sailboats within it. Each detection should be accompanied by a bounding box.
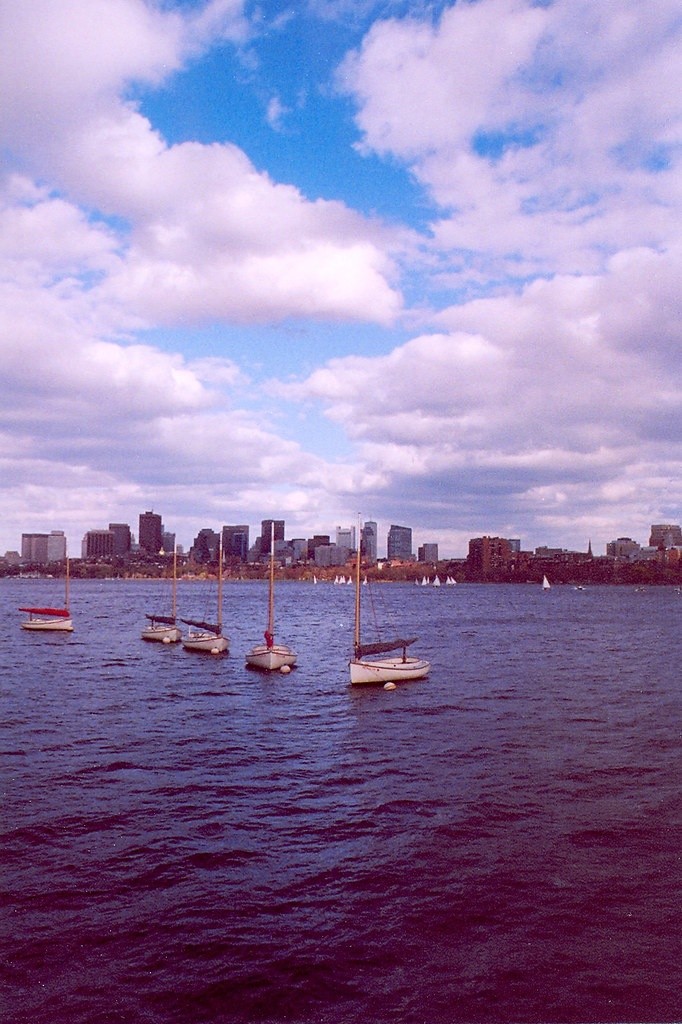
[363,575,368,586]
[422,576,430,586]
[415,578,419,586]
[245,521,298,671]
[313,575,317,585]
[339,574,345,584]
[18,556,73,630]
[178,532,231,652]
[445,576,457,586]
[542,575,550,590]
[141,538,182,642]
[347,574,352,585]
[333,574,339,584]
[348,512,431,685]
[432,574,440,587]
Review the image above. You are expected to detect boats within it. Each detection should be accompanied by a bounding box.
[674,584,682,593]
[577,583,583,590]
[635,586,647,592]
